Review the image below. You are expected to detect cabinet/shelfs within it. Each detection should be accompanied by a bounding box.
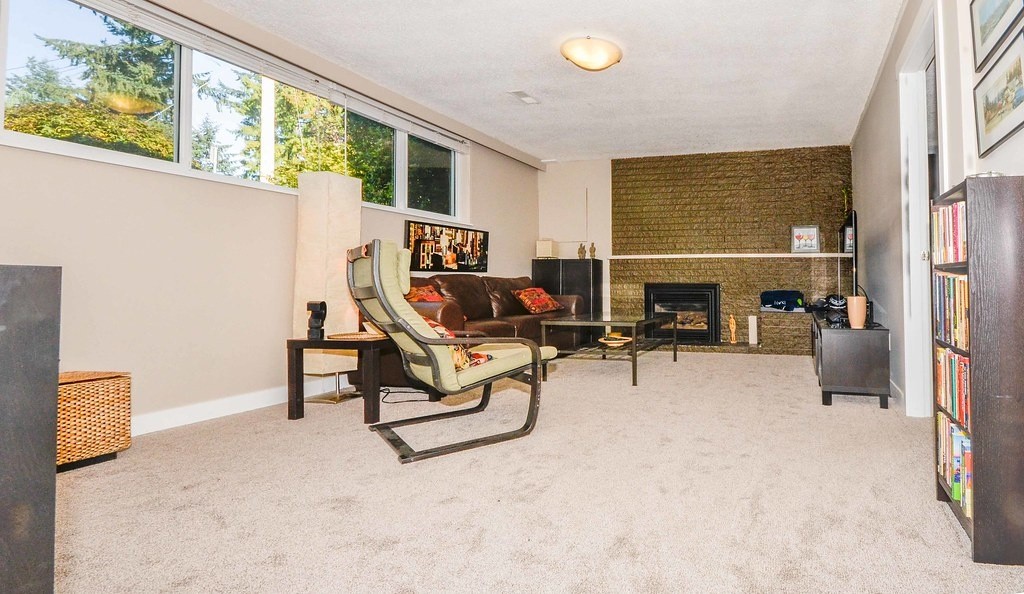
[929,177,1024,566]
[811,311,890,409]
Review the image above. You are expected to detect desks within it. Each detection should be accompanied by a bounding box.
[533,258,603,347]
[287,337,398,424]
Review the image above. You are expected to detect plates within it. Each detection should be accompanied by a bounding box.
[598,337,632,346]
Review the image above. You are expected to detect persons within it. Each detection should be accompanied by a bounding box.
[728,314,736,342]
[590,243,596,259]
[578,243,586,259]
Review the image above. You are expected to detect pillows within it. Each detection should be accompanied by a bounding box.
[420,313,492,371]
[406,284,466,320]
[510,288,563,314]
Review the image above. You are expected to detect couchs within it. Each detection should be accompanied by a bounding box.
[357,274,585,386]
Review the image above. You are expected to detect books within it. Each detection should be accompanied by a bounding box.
[930,270,969,353]
[931,201,969,267]
[935,344,971,434]
[937,412,974,519]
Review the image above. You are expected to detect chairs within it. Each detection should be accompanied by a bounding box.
[346,246,557,461]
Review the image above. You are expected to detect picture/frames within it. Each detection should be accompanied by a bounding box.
[969,0,1024,74]
[973,28,1024,158]
[405,220,489,273]
[791,225,821,253]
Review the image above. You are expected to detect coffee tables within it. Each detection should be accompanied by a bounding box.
[541,313,678,389]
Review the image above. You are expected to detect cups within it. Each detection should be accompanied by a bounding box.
[847,297,866,329]
[608,332,622,337]
